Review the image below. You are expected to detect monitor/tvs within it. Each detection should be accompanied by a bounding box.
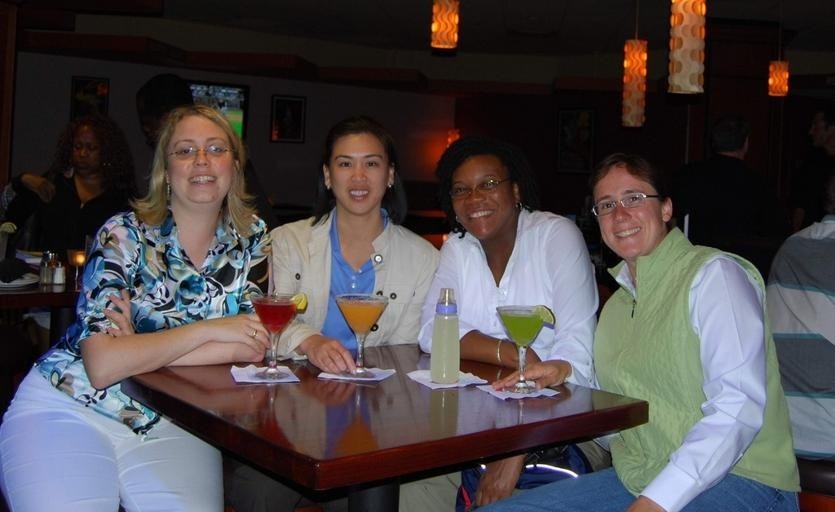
[182,78,250,141]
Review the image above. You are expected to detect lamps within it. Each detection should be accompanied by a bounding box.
[767,0,790,98]
[621,0,649,128]
[430,0,461,50]
[667,0,708,95]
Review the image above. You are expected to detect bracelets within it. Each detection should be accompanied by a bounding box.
[497,339,504,368]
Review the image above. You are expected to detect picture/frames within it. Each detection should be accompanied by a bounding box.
[556,105,598,177]
[269,94,307,144]
[69,75,110,127]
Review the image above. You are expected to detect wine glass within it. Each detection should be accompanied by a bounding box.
[337,294,390,378]
[494,304,548,393]
[248,292,299,381]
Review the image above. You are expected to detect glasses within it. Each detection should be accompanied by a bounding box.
[445,176,513,200]
[590,191,663,218]
[165,143,237,161]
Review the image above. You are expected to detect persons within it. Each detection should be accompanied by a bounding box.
[785,104,835,235]
[766,170,835,463]
[224,117,439,512]
[400,133,610,512]
[0,112,141,374]
[0,105,271,512]
[128,74,281,227]
[664,113,776,265]
[469,152,803,512]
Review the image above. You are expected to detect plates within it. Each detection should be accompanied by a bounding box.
[0,272,39,287]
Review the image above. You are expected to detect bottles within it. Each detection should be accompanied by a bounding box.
[40,250,67,285]
[432,287,461,385]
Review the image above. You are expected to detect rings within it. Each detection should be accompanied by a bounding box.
[250,329,257,338]
